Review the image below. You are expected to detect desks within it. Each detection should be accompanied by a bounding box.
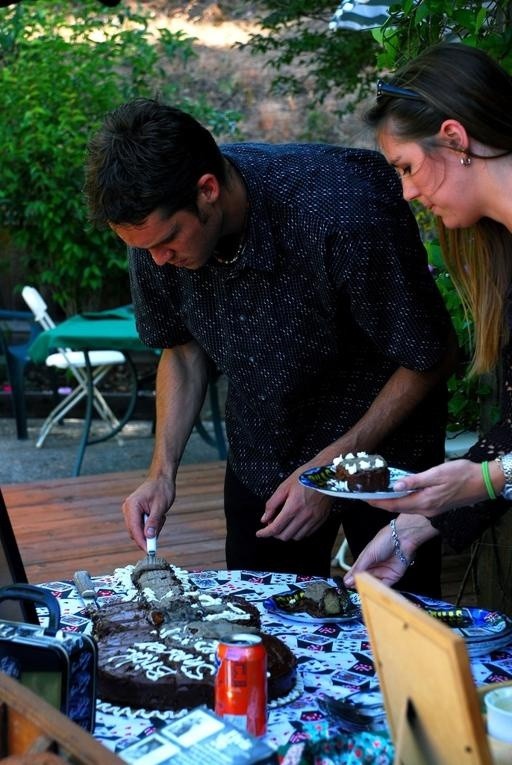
[25,301,227,478]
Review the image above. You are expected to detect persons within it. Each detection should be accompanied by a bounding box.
[331,40,511,599]
[83,90,463,600]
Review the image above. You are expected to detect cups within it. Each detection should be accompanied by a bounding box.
[484,685,512,744]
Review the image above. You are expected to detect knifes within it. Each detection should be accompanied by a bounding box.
[73,570,101,618]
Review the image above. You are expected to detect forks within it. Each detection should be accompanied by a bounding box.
[315,693,386,732]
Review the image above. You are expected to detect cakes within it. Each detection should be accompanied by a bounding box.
[91,590,298,712]
[333,451,390,493]
[304,580,344,618]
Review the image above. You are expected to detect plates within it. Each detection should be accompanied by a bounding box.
[430,606,511,658]
[264,590,365,624]
[299,464,420,500]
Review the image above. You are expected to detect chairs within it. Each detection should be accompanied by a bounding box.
[25,286,125,447]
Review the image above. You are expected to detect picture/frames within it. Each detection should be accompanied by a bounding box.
[355,571,491,764]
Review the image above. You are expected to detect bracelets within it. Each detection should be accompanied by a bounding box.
[481,459,497,502]
[389,517,414,570]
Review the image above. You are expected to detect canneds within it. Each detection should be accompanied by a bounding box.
[214,633,268,740]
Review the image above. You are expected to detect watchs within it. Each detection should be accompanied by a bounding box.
[496,452,511,502]
